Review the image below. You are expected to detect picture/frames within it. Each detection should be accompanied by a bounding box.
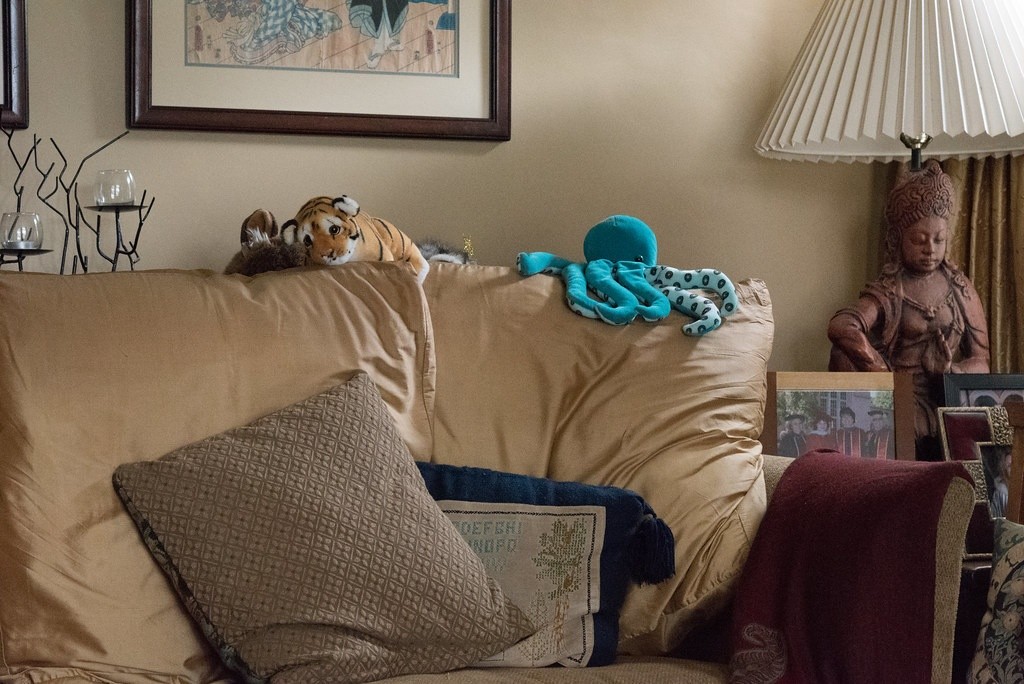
[938,368,1023,411]
[123,0,512,142]
[0,0,30,130]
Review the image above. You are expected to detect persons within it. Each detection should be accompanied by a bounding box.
[987,443,1014,521]
[777,407,894,461]
[826,160,992,452]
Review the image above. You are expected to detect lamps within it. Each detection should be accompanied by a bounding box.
[0,259,1023,684]
[751,0,1024,173]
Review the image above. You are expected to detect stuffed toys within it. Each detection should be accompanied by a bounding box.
[224,208,327,276]
[280,194,429,285]
[514,214,739,338]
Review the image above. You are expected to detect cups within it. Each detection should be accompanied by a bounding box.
[94,169,136,206]
[0,212,45,251]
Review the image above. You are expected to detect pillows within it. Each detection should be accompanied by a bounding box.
[405,456,678,666]
[109,374,535,684]
